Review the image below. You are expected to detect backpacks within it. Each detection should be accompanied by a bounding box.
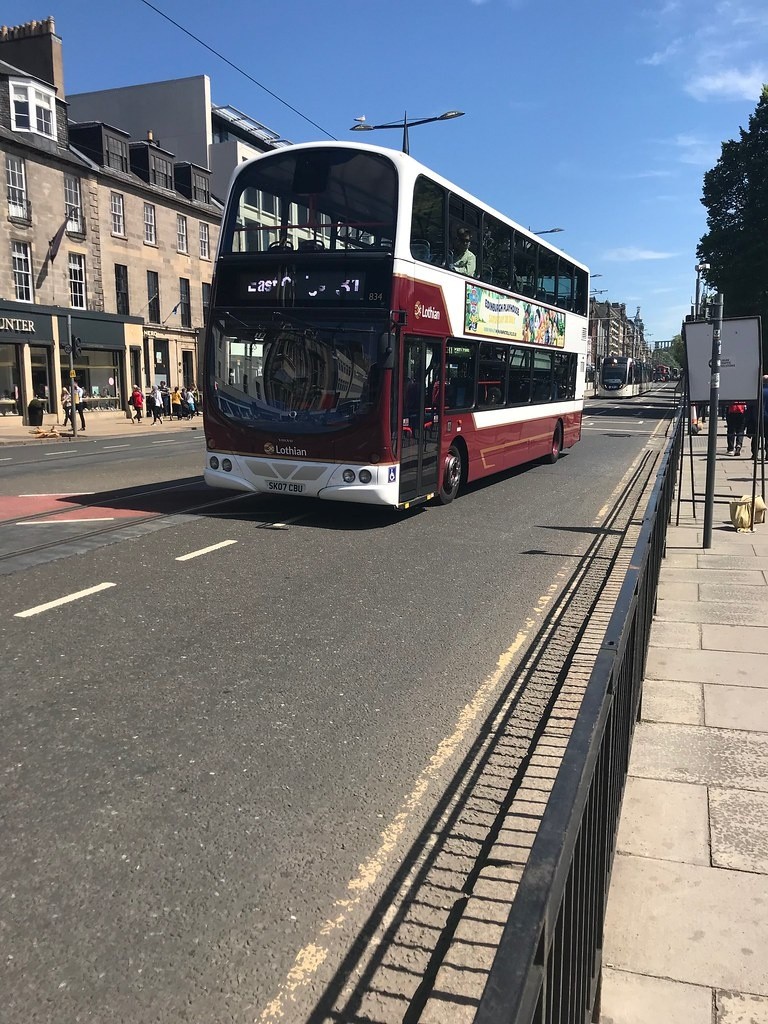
[128,393,134,405]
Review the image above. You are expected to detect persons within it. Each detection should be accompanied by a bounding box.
[180,388,191,421]
[149,384,164,425]
[170,386,184,422]
[187,384,201,419]
[696,404,707,422]
[61,386,73,426]
[158,381,168,422]
[128,384,144,423]
[361,343,378,409]
[513,265,523,294]
[68,382,86,431]
[750,375,768,461]
[448,226,476,277]
[721,401,750,457]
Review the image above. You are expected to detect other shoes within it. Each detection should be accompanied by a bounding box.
[188,415,194,420]
[725,451,734,456]
[63,424,66,426]
[131,418,134,424]
[179,419,183,421]
[735,445,741,456]
[151,422,156,426]
[78,428,85,431]
[170,415,173,421]
[161,420,163,424]
[138,421,142,423]
[68,428,73,431]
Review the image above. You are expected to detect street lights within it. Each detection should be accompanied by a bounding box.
[693,263,711,320]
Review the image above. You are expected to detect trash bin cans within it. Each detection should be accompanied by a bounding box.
[28,399,44,426]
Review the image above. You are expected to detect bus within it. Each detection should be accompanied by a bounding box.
[199,137,596,514]
[596,356,654,399]
[654,365,671,382]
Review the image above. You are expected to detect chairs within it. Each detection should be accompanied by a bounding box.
[267,241,293,253]
[430,247,575,312]
[406,377,574,416]
[369,240,393,248]
[409,239,431,262]
[298,240,325,252]
